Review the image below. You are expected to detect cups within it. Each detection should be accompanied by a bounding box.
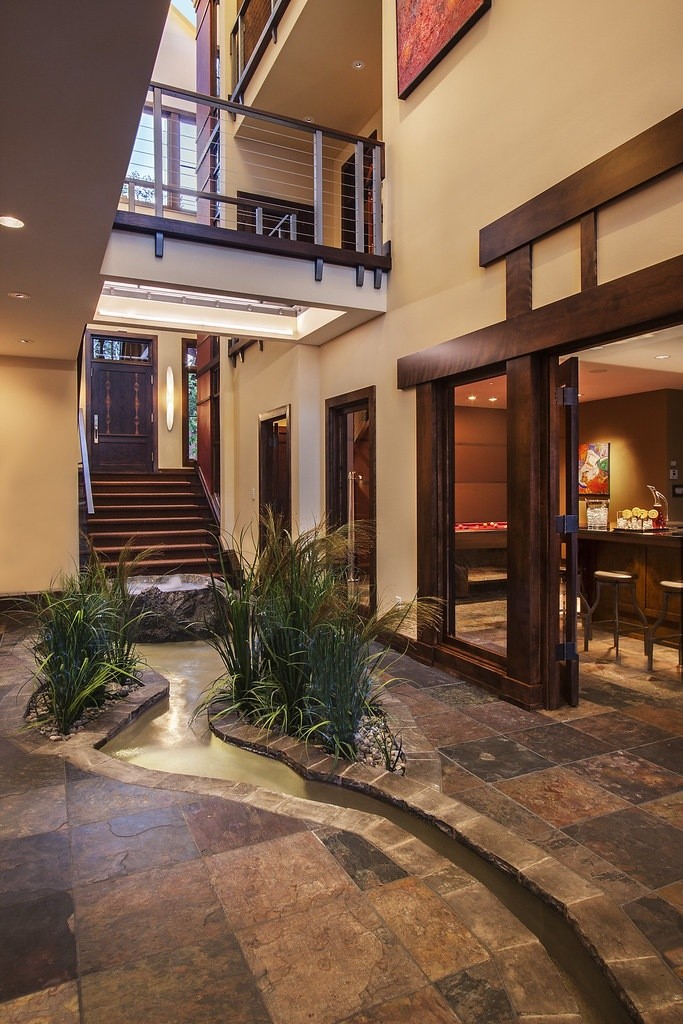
[631,512,642,530]
[643,511,653,530]
[617,511,629,528]
[587,508,608,531]
[653,504,665,529]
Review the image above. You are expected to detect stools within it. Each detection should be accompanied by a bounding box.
[647,580,683,671]
[557,566,592,641]
[584,571,651,666]
